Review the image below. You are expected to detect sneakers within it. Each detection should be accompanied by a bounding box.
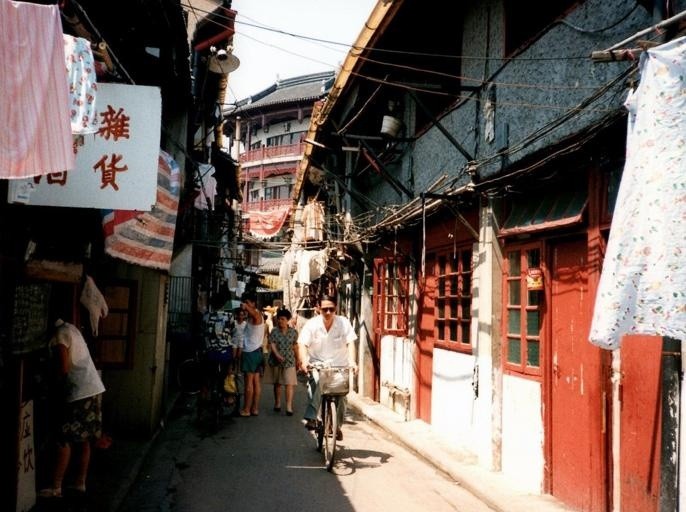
[234,410,251,418]
[335,430,344,441]
[251,411,260,417]
[273,405,282,412]
[286,408,294,417]
[304,419,316,429]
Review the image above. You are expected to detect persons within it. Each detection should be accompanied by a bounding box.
[232,312,246,374]
[240,292,265,416]
[39,309,107,500]
[297,294,359,440]
[202,292,235,382]
[268,309,299,416]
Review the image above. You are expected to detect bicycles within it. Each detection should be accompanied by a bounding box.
[313,363,350,470]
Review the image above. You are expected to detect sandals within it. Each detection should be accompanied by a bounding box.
[67,482,88,493]
[42,487,63,498]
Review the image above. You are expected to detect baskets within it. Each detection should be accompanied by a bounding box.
[317,366,351,398]
